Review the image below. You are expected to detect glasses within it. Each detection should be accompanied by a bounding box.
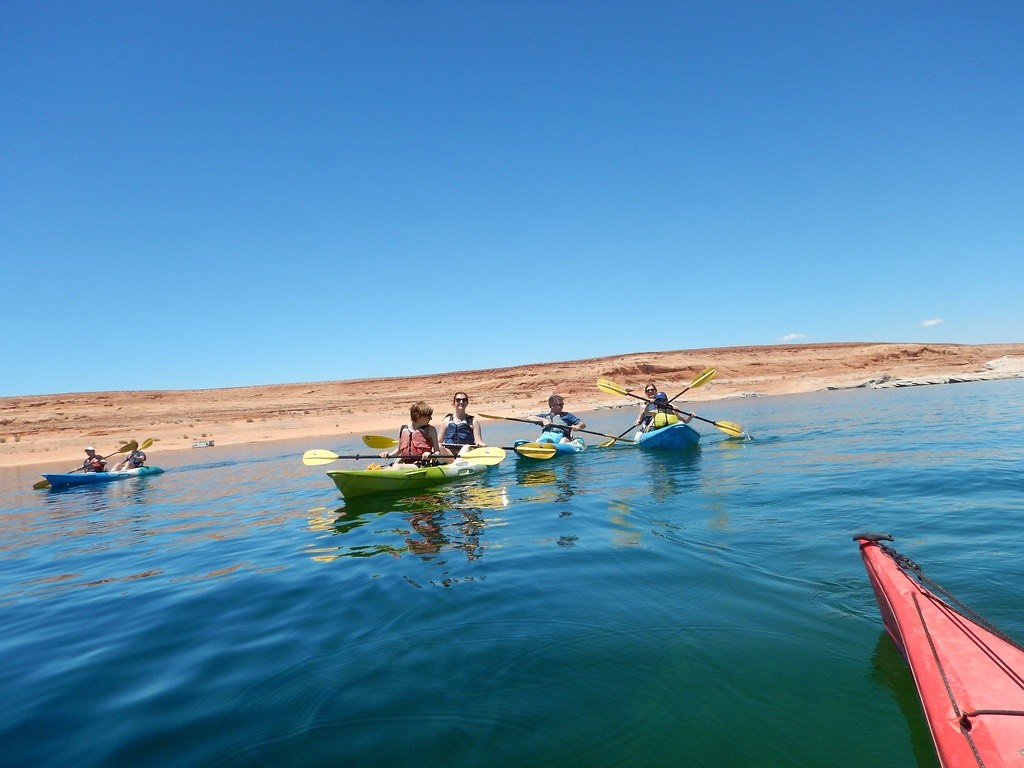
[656,398,666,402]
[553,403,564,406]
[454,398,468,402]
[646,388,656,392]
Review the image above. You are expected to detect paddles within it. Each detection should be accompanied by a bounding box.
[302,446,506,466]
[33,441,139,489]
[477,412,635,443]
[599,367,717,447]
[122,438,154,464]
[597,378,743,436]
[362,434,557,460]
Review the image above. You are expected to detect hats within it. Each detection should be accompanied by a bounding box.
[655,392,667,400]
[85,447,95,452]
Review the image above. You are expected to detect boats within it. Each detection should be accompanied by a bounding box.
[514,437,585,459]
[40,464,164,489]
[633,421,701,450]
[326,462,487,500]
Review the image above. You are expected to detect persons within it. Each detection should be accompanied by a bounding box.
[111,442,147,470]
[379,402,440,469]
[438,391,487,462]
[634,383,694,431]
[83,446,107,472]
[527,394,586,444]
